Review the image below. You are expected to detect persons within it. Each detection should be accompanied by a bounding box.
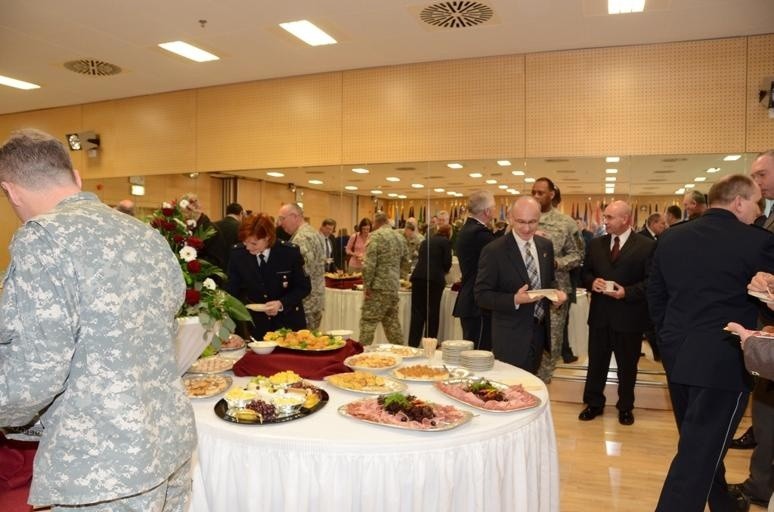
[529,177,586,386]
[718,270,773,507]
[472,196,568,375]
[748,149,774,234]
[752,197,768,228]
[112,200,133,216]
[407,224,453,348]
[450,189,500,351]
[645,173,773,511]
[358,208,413,347]
[177,185,712,282]
[278,202,327,330]
[578,200,656,425]
[0,127,199,512]
[224,211,312,333]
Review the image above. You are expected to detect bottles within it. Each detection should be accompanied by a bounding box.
[400,274,412,289]
[352,284,363,290]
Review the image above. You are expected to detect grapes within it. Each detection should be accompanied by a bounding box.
[246,400,275,420]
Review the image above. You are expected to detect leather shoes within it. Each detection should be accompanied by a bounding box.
[579,407,603,420]
[618,411,634,425]
[564,356,578,363]
[730,426,756,448]
[727,483,769,512]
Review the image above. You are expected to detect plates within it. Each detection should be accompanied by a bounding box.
[731,330,774,340]
[435,339,541,412]
[525,289,555,295]
[181,331,353,425]
[747,290,774,303]
[324,345,473,432]
[245,304,269,312]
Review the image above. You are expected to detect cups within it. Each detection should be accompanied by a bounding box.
[326,258,333,264]
[423,338,436,357]
[605,281,615,292]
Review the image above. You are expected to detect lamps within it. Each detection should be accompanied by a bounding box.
[66,130,99,151]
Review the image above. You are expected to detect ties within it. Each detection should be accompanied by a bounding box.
[612,237,620,258]
[259,253,266,266]
[325,239,329,258]
[526,241,544,320]
